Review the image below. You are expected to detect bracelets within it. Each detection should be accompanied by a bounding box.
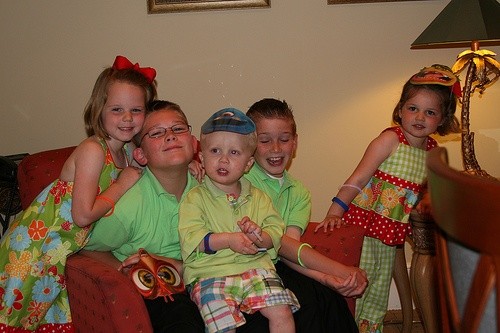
[338,184,362,194]
[96,194,114,217]
[331,197,349,212]
[297,242,312,268]
[203,233,217,254]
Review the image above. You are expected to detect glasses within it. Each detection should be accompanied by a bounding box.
[139,124,192,147]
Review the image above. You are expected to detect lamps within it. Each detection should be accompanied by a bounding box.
[410,0,500,179]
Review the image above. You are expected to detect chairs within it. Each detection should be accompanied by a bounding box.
[425,146,500,332]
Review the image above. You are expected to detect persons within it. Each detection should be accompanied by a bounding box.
[0,56,370,333]
[314,64,461,333]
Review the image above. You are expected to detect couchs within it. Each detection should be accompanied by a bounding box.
[18,145,365,333]
